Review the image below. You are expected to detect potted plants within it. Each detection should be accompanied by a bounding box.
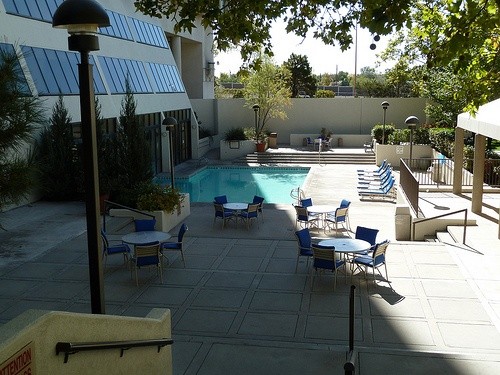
[255,135,268,153]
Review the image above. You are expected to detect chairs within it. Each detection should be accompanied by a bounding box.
[306,135,334,150]
[100,214,189,288]
[363,141,373,152]
[214,194,264,231]
[291,198,390,293]
[357,160,398,202]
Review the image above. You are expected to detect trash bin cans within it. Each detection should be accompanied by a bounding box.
[269,132,278,148]
[431,162,442,181]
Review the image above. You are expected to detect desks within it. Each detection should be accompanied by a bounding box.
[223,202,249,226]
[319,237,371,286]
[122,231,171,268]
[306,204,338,234]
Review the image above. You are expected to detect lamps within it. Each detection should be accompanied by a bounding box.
[192,124,196,130]
[162,131,167,137]
